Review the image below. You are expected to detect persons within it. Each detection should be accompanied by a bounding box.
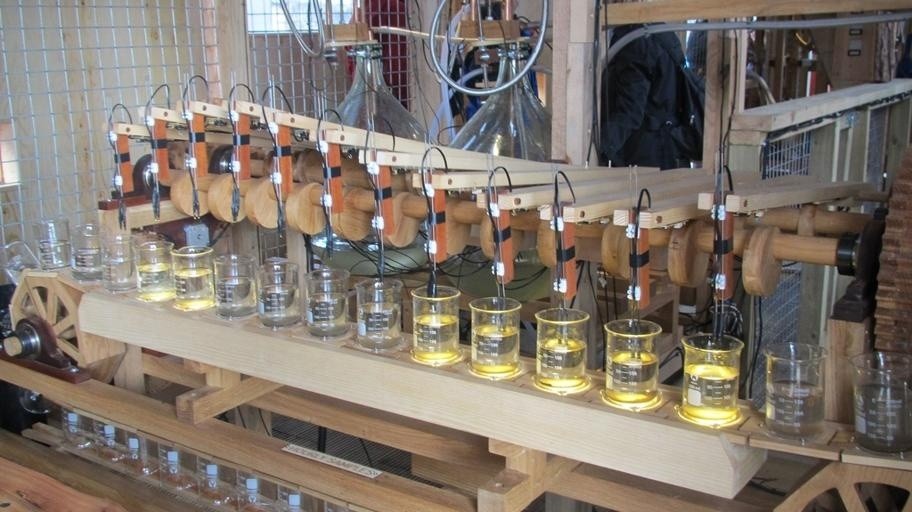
[461,0,551,159]
[686,19,780,179]
[600,0,692,170]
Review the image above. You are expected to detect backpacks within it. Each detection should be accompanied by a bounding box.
[653,34,703,161]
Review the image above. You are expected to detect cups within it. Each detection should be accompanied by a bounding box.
[254,256,300,333]
[598,316,664,409]
[32,214,72,269]
[25,386,314,510]
[1,239,40,284]
[170,244,216,305]
[350,276,405,351]
[847,347,912,454]
[757,337,832,443]
[64,223,105,280]
[209,250,255,321]
[97,232,137,294]
[532,306,591,392]
[299,266,349,337]
[131,238,174,298]
[408,281,463,365]
[672,330,748,428]
[465,293,525,376]
